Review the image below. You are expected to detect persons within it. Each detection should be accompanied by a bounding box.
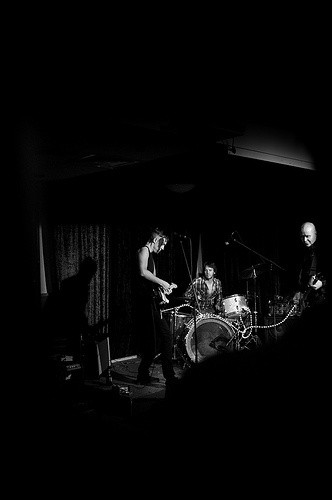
[128,226,180,385]
[185,262,222,313]
[291,222,332,295]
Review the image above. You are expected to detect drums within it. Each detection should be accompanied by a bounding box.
[222,295,250,320]
[177,316,234,365]
[163,312,193,337]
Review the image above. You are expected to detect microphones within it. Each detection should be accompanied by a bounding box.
[173,231,186,238]
[224,231,236,246]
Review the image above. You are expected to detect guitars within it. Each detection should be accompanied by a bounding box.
[153,282,178,304]
[296,271,323,317]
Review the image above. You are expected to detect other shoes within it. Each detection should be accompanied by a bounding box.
[165,376,181,382]
[137,376,159,385]
